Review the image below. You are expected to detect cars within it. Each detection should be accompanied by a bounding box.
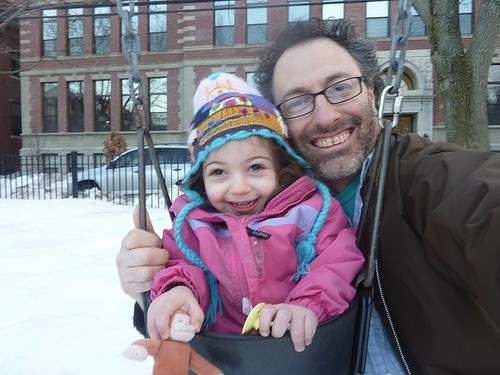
[59,142,193,196]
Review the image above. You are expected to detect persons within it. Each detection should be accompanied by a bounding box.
[139,72,365,354]
[114,16,500,375]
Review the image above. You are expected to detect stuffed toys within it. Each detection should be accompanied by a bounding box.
[124,310,225,375]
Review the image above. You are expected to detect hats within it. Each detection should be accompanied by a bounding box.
[173,72,332,329]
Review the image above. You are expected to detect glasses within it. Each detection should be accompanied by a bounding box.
[274,75,362,120]
